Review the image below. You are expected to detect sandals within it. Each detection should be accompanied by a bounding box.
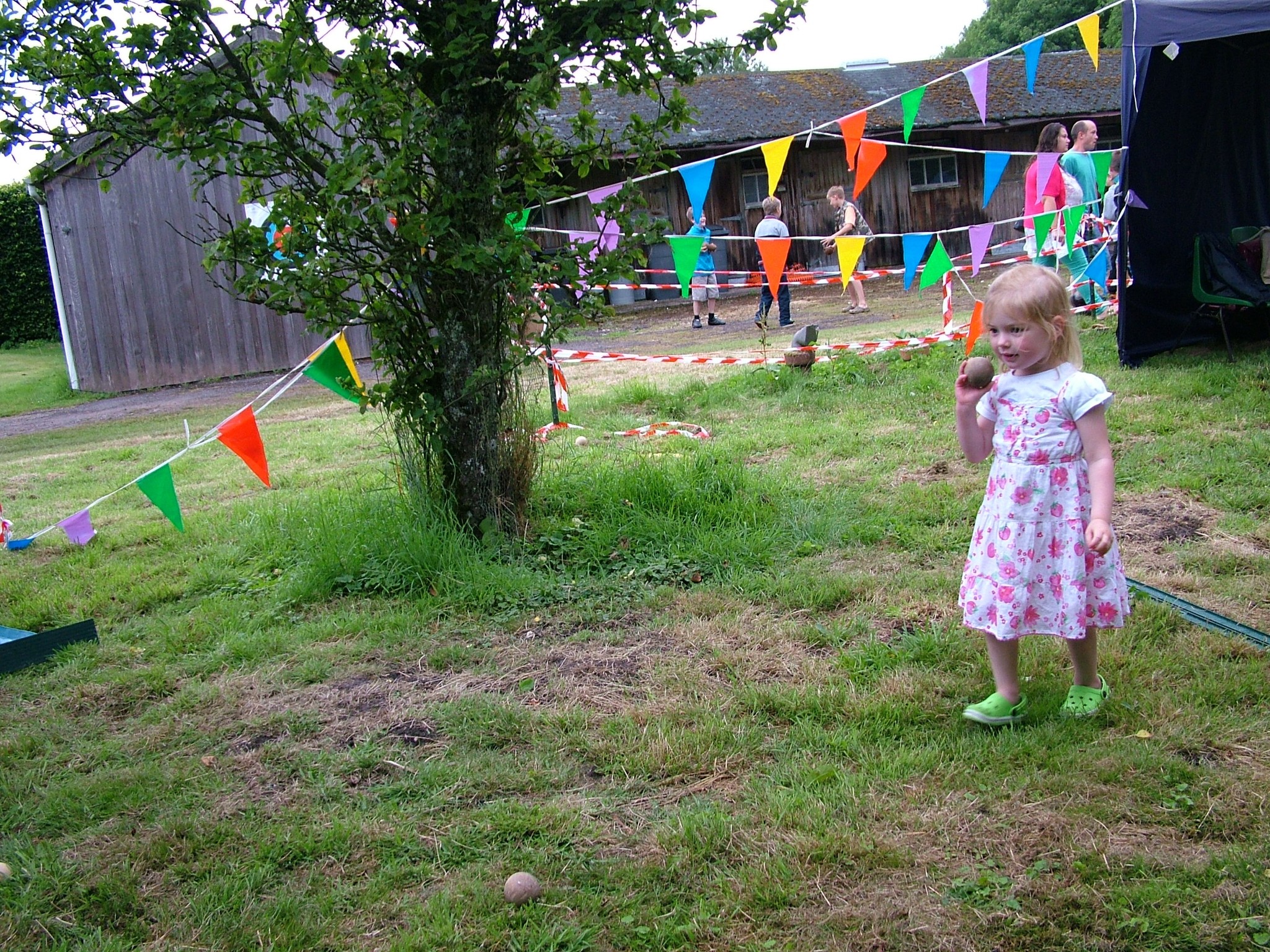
[962,691,1028,726]
[1061,673,1111,718]
[849,306,870,313]
[842,304,856,312]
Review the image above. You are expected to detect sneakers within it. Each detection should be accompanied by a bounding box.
[692,319,702,327]
[708,318,726,326]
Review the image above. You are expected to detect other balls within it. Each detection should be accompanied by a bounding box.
[576,437,587,447]
[701,243,708,249]
[964,357,994,389]
[504,872,539,905]
[824,247,834,255]
[710,243,719,251]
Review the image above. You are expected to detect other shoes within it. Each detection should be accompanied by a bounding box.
[1097,305,1119,319]
[756,320,769,329]
[1070,295,1092,316]
[781,320,795,328]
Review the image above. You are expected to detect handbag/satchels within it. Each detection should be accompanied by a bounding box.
[1013,211,1025,233]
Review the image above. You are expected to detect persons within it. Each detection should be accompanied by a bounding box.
[685,206,727,328]
[820,186,875,313]
[1023,123,1117,321]
[752,196,795,330]
[1058,120,1107,316]
[953,263,1132,724]
[1099,150,1133,296]
